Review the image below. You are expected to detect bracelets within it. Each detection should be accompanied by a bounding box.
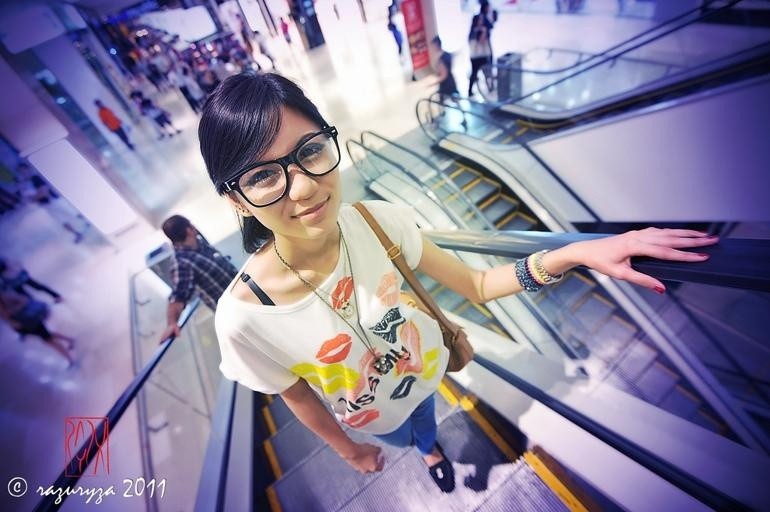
[513,248,565,293]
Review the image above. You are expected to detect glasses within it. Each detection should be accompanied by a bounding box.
[219,125,341,208]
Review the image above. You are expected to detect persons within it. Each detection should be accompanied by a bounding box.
[0,285,77,370]
[477,1,498,45]
[89,13,296,151]
[0,255,63,306]
[195,72,722,495]
[157,214,242,346]
[388,21,406,58]
[15,163,89,244]
[425,33,468,129]
[465,12,496,97]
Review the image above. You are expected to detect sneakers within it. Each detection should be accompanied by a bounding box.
[421,440,456,493]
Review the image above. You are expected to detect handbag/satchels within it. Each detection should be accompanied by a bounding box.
[353,201,475,373]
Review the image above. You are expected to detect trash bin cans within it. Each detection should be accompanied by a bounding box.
[498,53,522,102]
[145,243,175,291]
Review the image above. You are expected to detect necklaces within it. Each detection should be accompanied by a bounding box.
[269,220,387,371]
